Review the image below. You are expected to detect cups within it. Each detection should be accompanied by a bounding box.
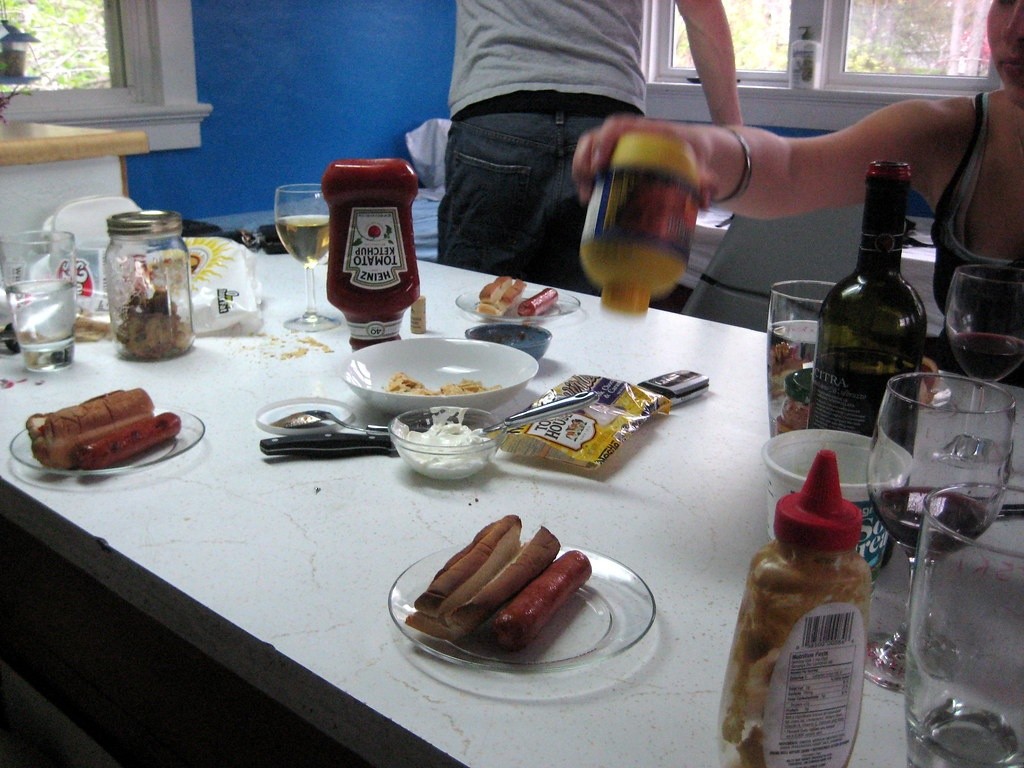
[769,279,838,438]
[411,296,426,333]
[0,231,77,371]
[906,483,1024,768]
[763,428,912,601]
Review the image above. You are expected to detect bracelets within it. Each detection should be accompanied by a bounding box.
[705,125,752,205]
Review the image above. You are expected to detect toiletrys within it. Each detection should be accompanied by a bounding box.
[788,26,822,90]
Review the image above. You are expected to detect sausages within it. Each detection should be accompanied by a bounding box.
[490,549,594,654]
[517,288,558,316]
[76,412,182,469]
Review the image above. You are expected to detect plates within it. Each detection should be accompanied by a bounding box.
[455,289,581,324]
[388,542,657,673]
[10,406,205,473]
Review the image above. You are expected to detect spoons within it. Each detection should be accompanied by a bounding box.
[270,410,391,441]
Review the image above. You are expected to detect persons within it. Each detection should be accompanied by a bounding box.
[573,0,1024,388]
[437,0,742,297]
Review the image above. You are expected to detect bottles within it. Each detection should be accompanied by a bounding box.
[105,209,196,361]
[716,450,871,768]
[323,159,419,351]
[811,160,928,457]
[578,130,698,313]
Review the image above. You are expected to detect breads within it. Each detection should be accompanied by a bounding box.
[477,275,526,317]
[27,387,155,469]
[405,515,561,644]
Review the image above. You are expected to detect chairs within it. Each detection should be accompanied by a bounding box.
[680,205,862,335]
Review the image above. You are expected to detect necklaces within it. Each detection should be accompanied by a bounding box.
[1013,104,1024,157]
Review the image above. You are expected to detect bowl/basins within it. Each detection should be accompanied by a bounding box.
[389,406,507,481]
[464,325,553,360]
[337,337,539,417]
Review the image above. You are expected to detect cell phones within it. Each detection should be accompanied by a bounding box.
[636,370,711,407]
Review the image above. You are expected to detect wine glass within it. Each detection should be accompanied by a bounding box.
[938,266,1024,470]
[862,372,1016,692]
[274,183,336,331]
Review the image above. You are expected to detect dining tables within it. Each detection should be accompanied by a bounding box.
[0,251,1024,768]
